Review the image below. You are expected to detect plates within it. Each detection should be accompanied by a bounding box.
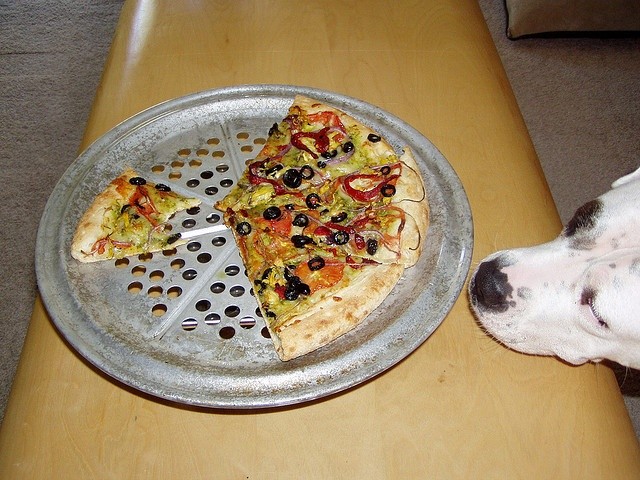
[35,82,474,407]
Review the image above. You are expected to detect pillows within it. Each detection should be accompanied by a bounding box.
[505,1,639,39]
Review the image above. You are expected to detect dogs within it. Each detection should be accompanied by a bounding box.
[465,169,640,369]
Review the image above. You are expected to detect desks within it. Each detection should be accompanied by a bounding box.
[0,0,639,480]
